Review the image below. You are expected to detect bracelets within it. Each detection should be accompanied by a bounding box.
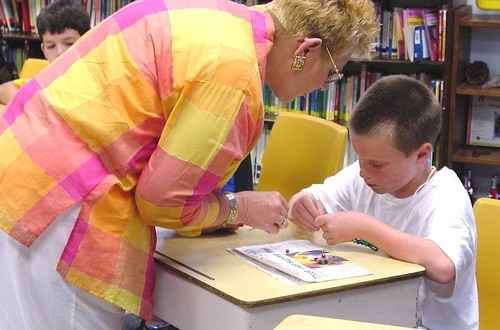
[222,192,238,227]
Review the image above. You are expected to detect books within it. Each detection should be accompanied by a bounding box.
[263,76,357,120]
[360,62,443,105]
[467,95,500,147]
[368,0,447,62]
[0,0,133,34]
[12,48,30,74]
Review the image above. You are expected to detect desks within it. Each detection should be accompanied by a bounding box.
[273,312,442,330]
[144,214,424,330]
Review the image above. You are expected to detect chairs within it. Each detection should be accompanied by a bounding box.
[460,196,500,330]
[253,110,351,200]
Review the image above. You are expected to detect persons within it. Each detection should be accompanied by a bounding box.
[288,74,479,330]
[0,0,91,106]
[0,0,383,330]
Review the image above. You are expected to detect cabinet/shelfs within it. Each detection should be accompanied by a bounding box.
[0,0,500,204]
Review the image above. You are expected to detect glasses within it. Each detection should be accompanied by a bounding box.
[321,42,344,83]
